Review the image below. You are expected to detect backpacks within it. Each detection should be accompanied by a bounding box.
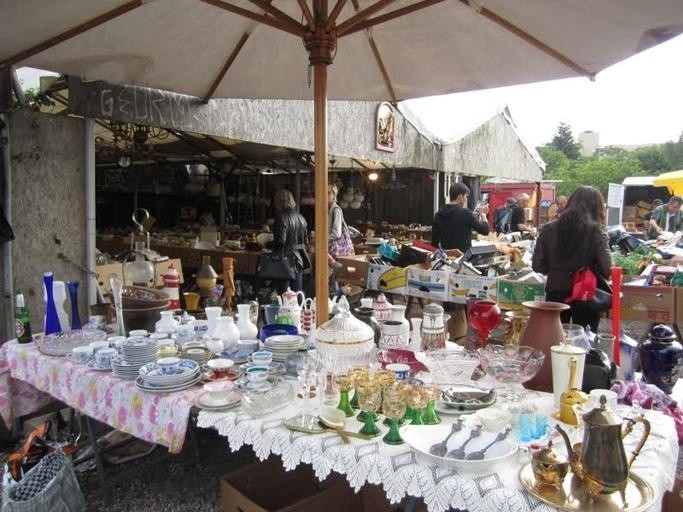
[493,207,512,236]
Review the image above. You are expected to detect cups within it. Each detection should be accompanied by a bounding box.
[588,389,617,414]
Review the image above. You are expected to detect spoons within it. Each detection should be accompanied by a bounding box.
[464,426,512,460]
[429,418,465,457]
[446,425,484,460]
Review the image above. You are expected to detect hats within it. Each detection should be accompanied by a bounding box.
[563,266,597,304]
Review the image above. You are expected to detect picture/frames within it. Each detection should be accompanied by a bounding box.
[375,101,396,152]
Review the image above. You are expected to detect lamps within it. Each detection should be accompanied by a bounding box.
[378,164,407,190]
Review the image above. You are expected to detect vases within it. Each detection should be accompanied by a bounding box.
[521,301,571,393]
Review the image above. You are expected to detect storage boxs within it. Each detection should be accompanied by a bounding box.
[220,455,363,512]
[623,200,652,230]
[333,248,547,305]
[609,284,675,324]
[363,477,413,511]
[675,287,683,327]
[96,235,270,294]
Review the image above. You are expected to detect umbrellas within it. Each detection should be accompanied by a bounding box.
[0,1,683,326]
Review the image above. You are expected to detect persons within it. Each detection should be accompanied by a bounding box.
[309,184,356,257]
[269,189,312,292]
[431,182,490,253]
[508,192,536,238]
[306,252,352,302]
[494,197,516,234]
[531,184,612,332]
[647,195,683,241]
[643,198,664,230]
[553,195,567,218]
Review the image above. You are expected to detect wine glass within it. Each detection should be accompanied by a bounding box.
[332,363,442,445]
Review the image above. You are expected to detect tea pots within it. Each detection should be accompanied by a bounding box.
[304,295,339,334]
[550,338,587,401]
[554,394,650,508]
[276,287,304,332]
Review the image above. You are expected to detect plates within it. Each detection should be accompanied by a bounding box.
[399,424,518,472]
[71,301,308,411]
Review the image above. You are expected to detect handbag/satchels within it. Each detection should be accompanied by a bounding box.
[579,264,612,311]
[255,255,294,280]
[328,231,354,256]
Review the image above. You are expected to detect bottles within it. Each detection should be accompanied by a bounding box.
[14,289,32,343]
